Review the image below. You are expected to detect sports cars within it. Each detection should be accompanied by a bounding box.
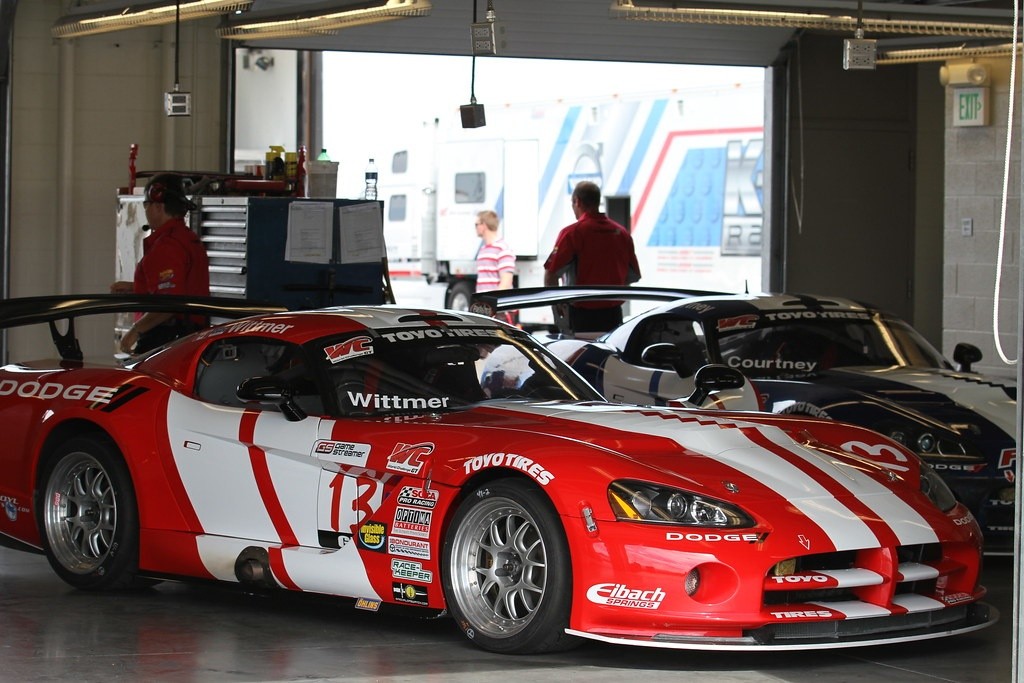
[479,288,1017,559]
[0,290,1000,656]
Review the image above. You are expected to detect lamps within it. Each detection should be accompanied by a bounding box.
[940,63,990,128]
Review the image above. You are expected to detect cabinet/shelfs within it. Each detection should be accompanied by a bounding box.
[113,195,384,341]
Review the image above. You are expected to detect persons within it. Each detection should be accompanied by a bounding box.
[473,211,517,324]
[111,173,212,360]
[543,181,642,334]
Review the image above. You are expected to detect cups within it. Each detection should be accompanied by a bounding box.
[307,161,339,199]
[243,165,266,196]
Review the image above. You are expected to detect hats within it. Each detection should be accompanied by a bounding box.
[142,172,199,214]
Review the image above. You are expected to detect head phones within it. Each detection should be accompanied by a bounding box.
[148,183,198,212]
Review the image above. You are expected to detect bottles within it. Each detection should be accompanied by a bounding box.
[365,158,378,200]
[313,147,331,161]
[285,151,297,192]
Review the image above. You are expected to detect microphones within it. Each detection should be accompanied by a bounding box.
[142,224,150,231]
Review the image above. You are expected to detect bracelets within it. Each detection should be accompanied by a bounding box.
[132,321,144,339]
